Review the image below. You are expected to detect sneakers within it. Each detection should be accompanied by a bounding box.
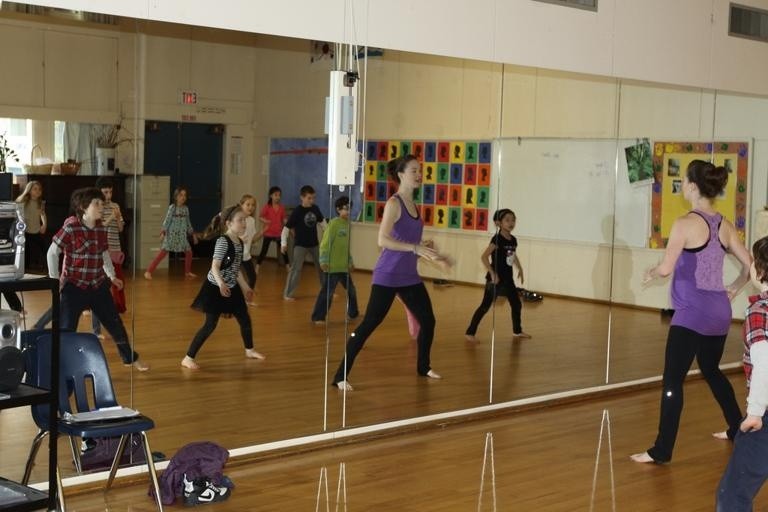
[80,437,97,454]
[182,474,230,507]
[522,291,543,301]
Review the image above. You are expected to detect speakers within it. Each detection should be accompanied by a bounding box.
[0,309,24,392]
[0,200,27,283]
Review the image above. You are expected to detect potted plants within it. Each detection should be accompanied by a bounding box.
[0,136,19,173]
[95,114,134,176]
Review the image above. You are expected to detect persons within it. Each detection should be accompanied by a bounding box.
[629,159,754,463]
[462,209,531,343]
[46,188,151,371]
[238,195,269,290]
[281,185,325,301]
[333,153,452,391]
[144,187,197,279]
[16,180,47,272]
[311,196,364,325]
[33,177,124,338]
[256,186,288,273]
[716,236,767,512]
[181,207,265,370]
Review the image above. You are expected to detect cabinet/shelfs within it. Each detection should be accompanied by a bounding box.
[0,274,59,512]
[136,176,170,269]
[15,174,139,273]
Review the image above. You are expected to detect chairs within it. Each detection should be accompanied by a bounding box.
[22,334,163,511]
[19,328,82,475]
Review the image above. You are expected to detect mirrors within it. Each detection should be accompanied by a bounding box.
[0,16,768,486]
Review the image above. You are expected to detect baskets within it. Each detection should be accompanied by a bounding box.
[24,144,53,174]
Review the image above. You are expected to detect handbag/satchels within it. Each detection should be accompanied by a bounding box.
[74,434,147,471]
[150,442,229,505]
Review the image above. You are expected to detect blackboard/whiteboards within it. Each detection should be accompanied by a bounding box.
[489,137,755,252]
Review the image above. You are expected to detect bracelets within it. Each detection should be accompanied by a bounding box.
[650,270,655,278]
[413,244,416,256]
